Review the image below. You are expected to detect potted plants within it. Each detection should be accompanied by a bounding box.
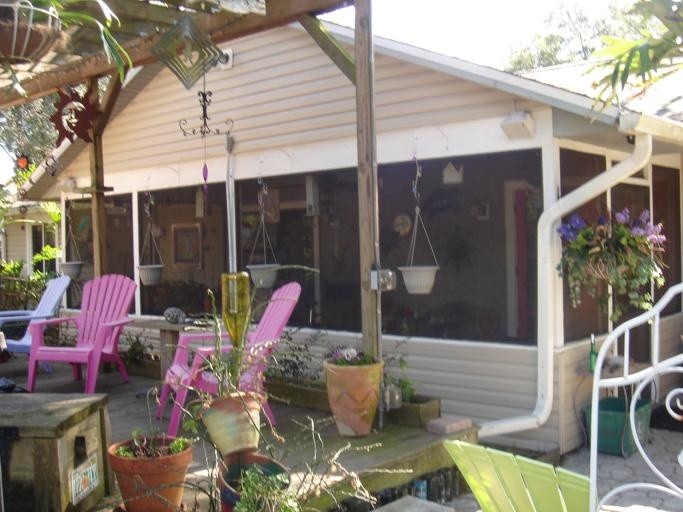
[217,456,292,511]
[196,391,267,457]
[0,0,132,99]
[326,347,384,437]
[108,432,194,512]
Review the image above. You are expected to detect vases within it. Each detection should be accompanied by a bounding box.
[139,265,164,286]
[397,266,440,295]
[61,262,84,278]
[246,264,280,288]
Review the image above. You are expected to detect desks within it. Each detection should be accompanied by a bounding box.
[0,393,115,512]
[573,363,657,460]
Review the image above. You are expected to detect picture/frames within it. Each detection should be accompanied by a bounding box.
[172,223,203,269]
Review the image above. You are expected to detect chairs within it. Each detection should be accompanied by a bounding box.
[155,282,302,437]
[26,274,137,393]
[443,439,599,512]
[0,276,71,374]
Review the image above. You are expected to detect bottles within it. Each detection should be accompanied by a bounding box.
[589,333,597,374]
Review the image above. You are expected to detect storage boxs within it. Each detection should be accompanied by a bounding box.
[581,398,654,456]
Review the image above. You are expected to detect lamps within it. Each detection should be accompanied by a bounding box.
[210,48,233,69]
[503,111,536,140]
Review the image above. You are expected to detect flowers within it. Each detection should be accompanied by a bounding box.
[556,206,670,325]
